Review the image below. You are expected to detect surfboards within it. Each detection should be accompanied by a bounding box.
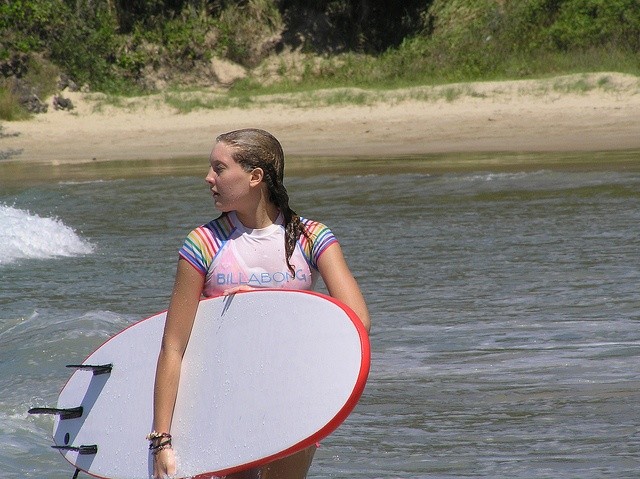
[28,288,371,479]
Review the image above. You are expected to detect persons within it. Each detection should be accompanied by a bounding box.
[145,128,371,478]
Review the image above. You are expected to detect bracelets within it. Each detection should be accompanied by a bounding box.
[146,432,173,454]
